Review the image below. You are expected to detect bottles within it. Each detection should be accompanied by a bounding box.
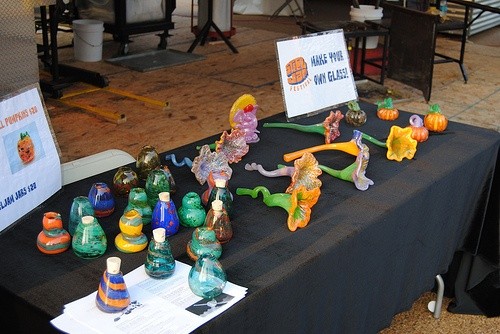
[88,182,114,217]
[179,191,206,227]
[71,215,107,259]
[188,253,227,299]
[17,131,35,163]
[68,196,94,237]
[145,228,175,280]
[36,211,70,254]
[209,179,232,214]
[96,257,130,314]
[152,191,179,237]
[205,199,232,244]
[123,188,152,225]
[112,167,138,198]
[136,145,175,207]
[115,210,147,253]
[427,0,448,22]
[187,227,222,260]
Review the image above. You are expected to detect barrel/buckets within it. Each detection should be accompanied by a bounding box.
[349,5,383,49]
[72,19,104,62]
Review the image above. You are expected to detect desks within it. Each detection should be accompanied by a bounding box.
[298,16,471,97]
[0,103,500,334]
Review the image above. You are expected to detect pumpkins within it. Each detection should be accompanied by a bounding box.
[344,101,367,127]
[423,104,448,132]
[377,98,398,121]
[407,115,428,141]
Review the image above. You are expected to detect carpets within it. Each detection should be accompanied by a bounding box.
[106,48,209,72]
